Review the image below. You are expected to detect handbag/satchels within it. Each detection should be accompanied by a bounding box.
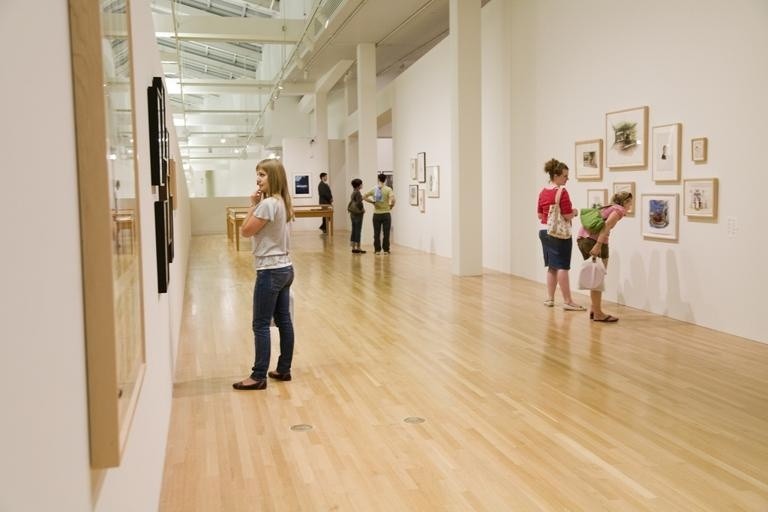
[546,185,573,240]
[347,191,366,215]
[579,203,613,234]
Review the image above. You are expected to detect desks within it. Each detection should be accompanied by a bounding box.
[225,205,334,250]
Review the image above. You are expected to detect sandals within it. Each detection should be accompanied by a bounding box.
[268,370,292,381]
[563,303,587,312]
[543,299,555,306]
[233,377,267,392]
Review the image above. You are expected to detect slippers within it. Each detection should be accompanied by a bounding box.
[590,311,619,322]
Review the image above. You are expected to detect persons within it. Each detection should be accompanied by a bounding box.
[538,159,587,311]
[114,180,121,247]
[576,191,632,322]
[363,174,395,255]
[318,173,333,233]
[351,179,366,253]
[233,159,295,390]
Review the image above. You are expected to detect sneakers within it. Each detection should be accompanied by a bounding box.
[351,249,366,254]
[374,250,391,255]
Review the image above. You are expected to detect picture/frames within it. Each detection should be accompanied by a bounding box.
[605,106,649,168]
[407,151,441,214]
[293,173,311,198]
[148,73,178,294]
[610,181,637,214]
[573,138,603,180]
[640,122,721,245]
[586,188,608,208]
[64,0,148,468]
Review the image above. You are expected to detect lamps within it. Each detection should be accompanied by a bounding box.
[266,11,330,112]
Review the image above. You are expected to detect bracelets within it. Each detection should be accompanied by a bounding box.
[251,204,255,209]
[595,242,603,246]
